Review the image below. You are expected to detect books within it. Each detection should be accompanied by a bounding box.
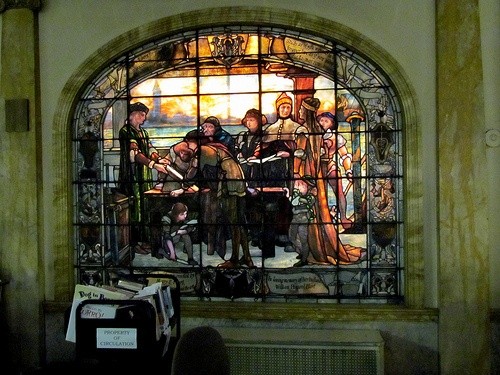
[65,278,175,342]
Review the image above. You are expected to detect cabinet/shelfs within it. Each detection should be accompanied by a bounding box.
[75,275,181,375]
